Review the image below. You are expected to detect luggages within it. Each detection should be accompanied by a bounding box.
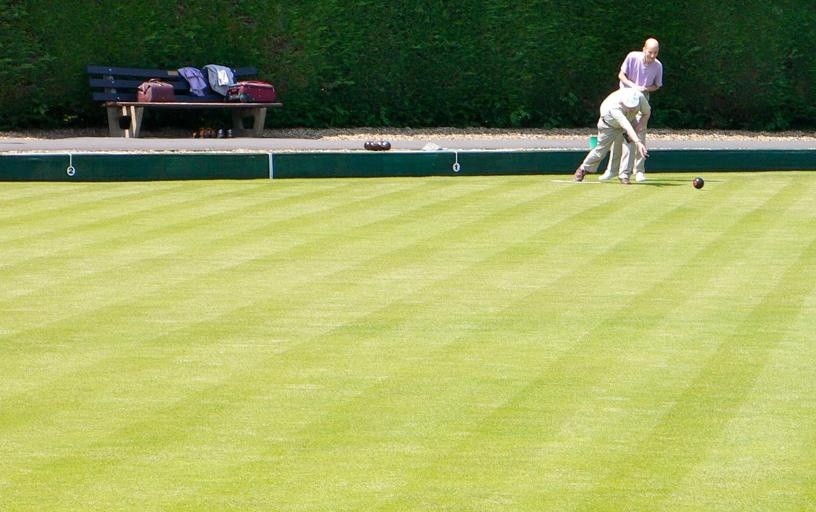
[227,80,275,103]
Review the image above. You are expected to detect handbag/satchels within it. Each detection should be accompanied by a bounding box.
[138,77,175,102]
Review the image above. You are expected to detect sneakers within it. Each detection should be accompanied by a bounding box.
[216,128,225,138]
[636,172,645,181]
[574,168,585,182]
[620,178,631,185]
[193,127,216,138]
[599,172,617,182]
[226,129,233,137]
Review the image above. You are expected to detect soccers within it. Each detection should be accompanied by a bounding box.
[693,178,704,188]
[365,141,391,151]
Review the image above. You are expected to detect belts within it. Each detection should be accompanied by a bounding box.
[601,118,613,128]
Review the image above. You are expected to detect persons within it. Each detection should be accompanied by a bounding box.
[574,87,651,185]
[598,38,662,183]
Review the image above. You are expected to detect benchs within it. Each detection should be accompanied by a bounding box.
[86,64,284,138]
